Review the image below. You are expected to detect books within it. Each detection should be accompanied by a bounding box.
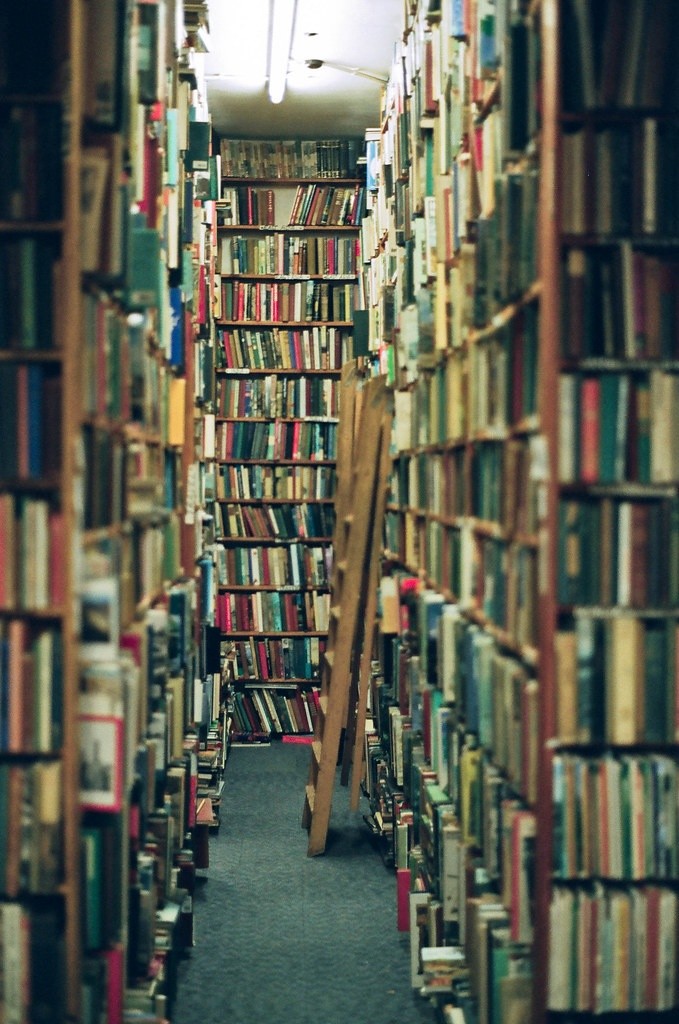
[365,1,678,1024]
[232,135,367,746]
[0,0,234,1023]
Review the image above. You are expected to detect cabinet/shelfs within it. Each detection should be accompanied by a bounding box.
[0,0,679,1024]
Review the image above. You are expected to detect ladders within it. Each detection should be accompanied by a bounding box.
[303,359,395,858]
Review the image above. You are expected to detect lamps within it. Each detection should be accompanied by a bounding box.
[268,0,297,105]
